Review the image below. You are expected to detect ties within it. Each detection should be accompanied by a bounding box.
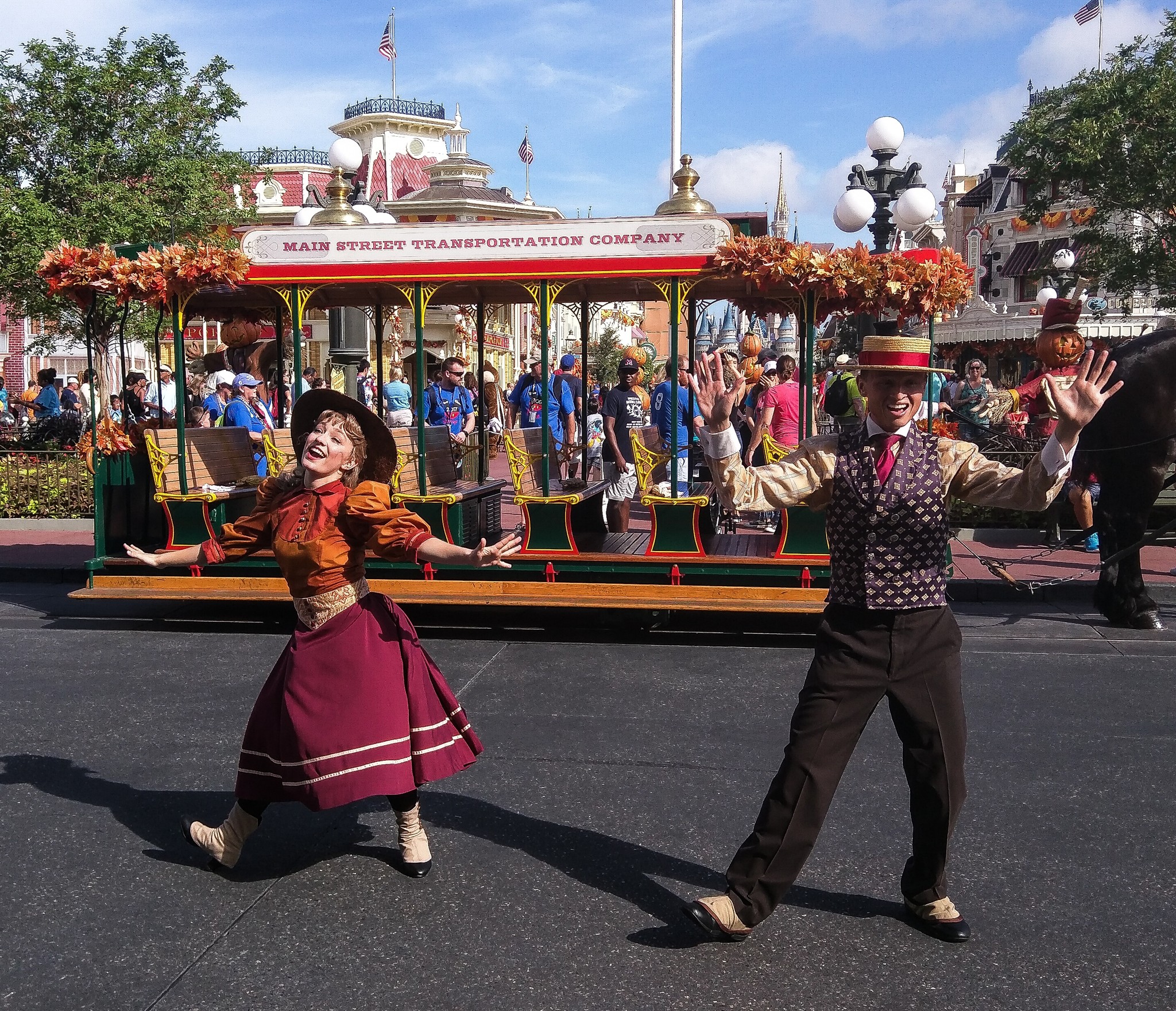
[870,434,903,486]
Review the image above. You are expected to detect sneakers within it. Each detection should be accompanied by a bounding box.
[1084,532,1100,555]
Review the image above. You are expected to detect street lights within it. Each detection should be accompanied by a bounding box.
[442,304,468,387]
[835,117,935,400]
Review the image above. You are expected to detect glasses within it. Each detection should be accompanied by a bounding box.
[160,369,169,373]
[241,384,257,389]
[678,368,689,374]
[309,373,317,381]
[765,371,777,376]
[530,361,541,367]
[444,370,465,377]
[969,366,981,370]
[223,383,233,389]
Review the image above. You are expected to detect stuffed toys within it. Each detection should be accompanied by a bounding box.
[483,360,506,460]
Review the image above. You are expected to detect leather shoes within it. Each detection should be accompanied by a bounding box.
[903,896,971,943]
[686,902,749,942]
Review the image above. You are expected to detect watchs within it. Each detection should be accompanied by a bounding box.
[461,430,469,437]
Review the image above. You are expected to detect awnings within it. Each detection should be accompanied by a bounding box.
[999,235,1095,278]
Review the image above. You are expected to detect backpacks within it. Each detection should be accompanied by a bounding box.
[214,401,254,427]
[825,376,856,416]
[468,388,488,421]
[267,387,278,420]
[357,377,369,406]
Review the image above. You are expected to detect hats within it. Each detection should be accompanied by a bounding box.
[291,389,398,483]
[834,354,852,366]
[67,377,79,384]
[132,364,264,389]
[523,349,642,371]
[764,360,777,373]
[754,348,776,365]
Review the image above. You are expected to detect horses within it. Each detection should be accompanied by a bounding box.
[1069,325,1176,632]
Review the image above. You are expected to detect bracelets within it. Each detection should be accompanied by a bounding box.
[960,400,963,404]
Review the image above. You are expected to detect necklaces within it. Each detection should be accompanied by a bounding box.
[236,395,259,418]
[216,392,223,413]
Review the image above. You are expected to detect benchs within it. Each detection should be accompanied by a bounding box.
[390,425,507,547]
[629,424,717,557]
[261,429,298,478]
[761,423,831,560]
[145,425,268,554]
[503,427,611,556]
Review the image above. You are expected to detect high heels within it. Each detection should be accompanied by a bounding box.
[405,858,432,878]
[181,819,239,878]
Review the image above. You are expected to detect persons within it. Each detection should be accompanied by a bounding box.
[688,335,1124,944]
[413,354,643,533]
[356,359,414,428]
[970,297,1084,438]
[0,364,270,477]
[651,352,1100,551]
[124,388,522,877]
[188,315,306,407]
[269,366,330,428]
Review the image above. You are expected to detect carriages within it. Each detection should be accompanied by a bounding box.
[64,219,1176,632]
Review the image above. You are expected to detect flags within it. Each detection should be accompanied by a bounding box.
[379,20,397,61]
[518,136,533,164]
